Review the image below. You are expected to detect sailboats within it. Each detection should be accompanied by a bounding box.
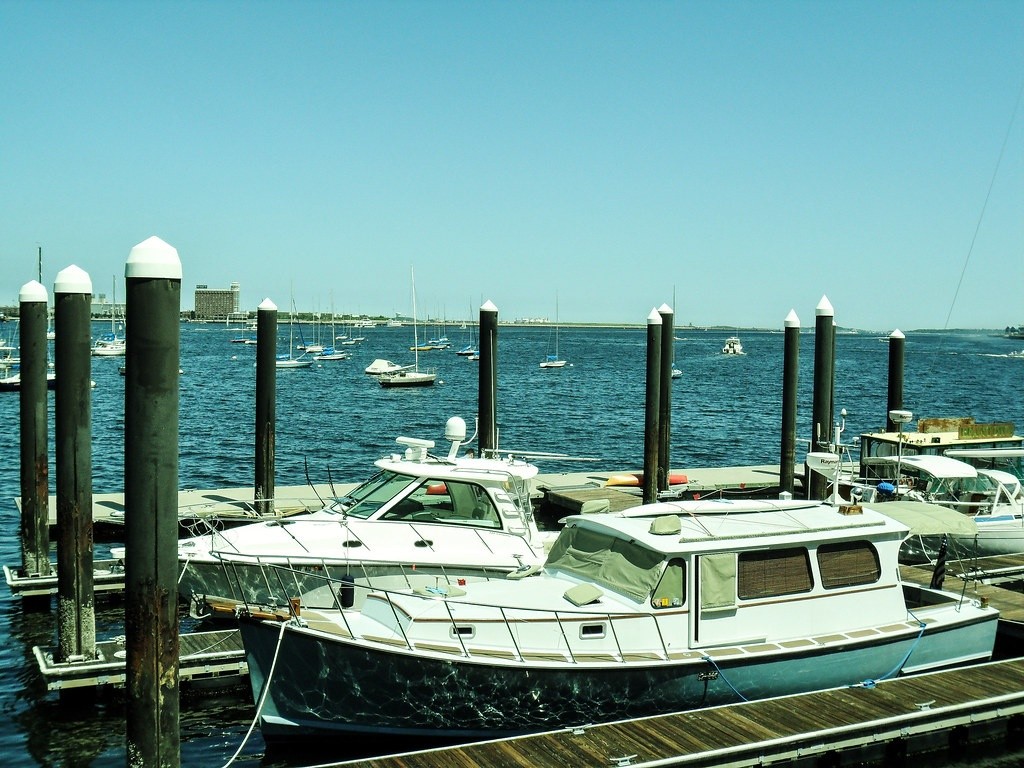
[538,295,567,369]
[89,274,126,357]
[671,284,683,379]
[376,267,439,388]
[230,292,480,369]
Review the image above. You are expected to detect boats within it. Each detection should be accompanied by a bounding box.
[1,245,55,392]
[828,410,1024,556]
[364,358,403,375]
[109,450,562,607]
[187,408,1001,739]
[722,336,742,355]
[371,415,540,481]
